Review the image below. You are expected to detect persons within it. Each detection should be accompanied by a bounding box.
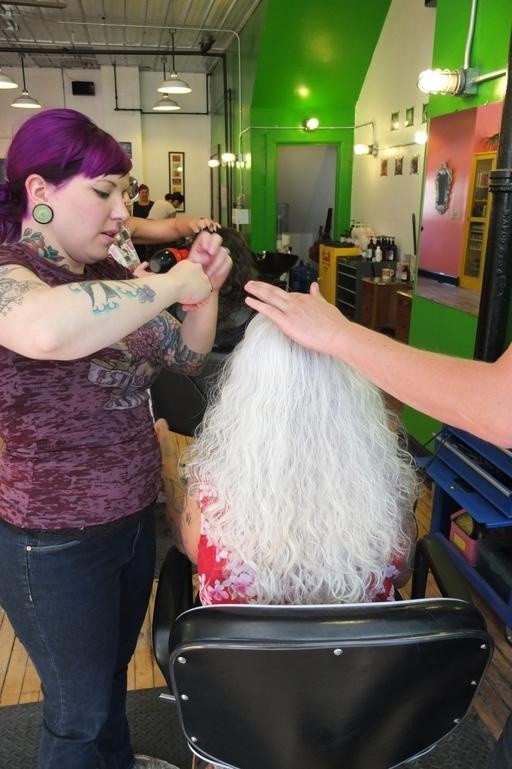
[110,176,220,281]
[147,191,184,220]
[0,109,234,769]
[243,280,511,453]
[176,227,254,351]
[131,184,154,219]
[155,311,415,607]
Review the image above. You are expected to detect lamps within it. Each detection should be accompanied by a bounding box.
[416,67,479,97]
[11,53,42,109]
[152,59,182,111]
[0,67,19,89]
[304,117,319,132]
[353,143,379,157]
[156,28,193,95]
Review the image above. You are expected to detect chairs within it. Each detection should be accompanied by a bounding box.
[151,536,493,769]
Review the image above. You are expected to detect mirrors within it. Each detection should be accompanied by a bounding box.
[416,100,502,318]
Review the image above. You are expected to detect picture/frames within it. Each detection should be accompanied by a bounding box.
[118,141,132,159]
[432,162,454,215]
[168,151,185,213]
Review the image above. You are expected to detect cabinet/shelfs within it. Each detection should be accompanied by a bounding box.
[458,149,497,290]
[414,424,512,633]
[334,255,397,325]
[360,276,412,344]
[318,238,363,320]
